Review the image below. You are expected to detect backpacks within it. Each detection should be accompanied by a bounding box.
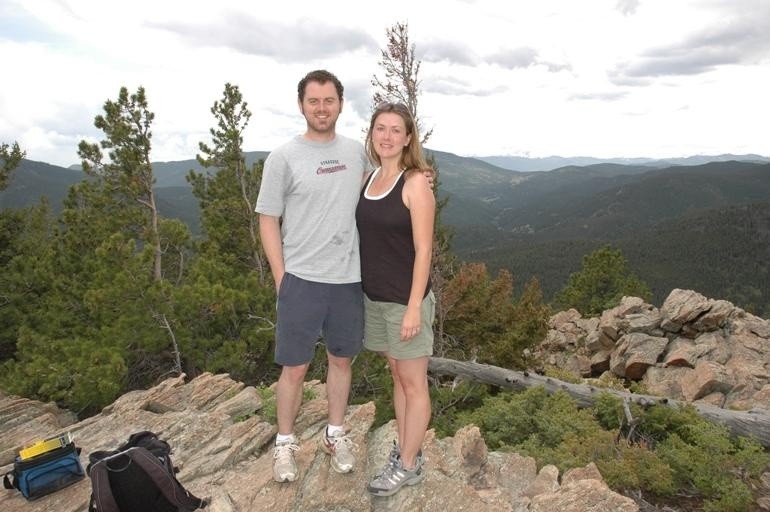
[87,431,208,512]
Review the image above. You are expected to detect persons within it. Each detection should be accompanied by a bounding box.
[354,99,436,495]
[254,70,433,484]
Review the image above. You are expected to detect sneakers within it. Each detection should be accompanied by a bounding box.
[367,439,426,497]
[273,437,301,484]
[321,425,361,475]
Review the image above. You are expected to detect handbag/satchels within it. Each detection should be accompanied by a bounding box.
[3,442,85,502]
